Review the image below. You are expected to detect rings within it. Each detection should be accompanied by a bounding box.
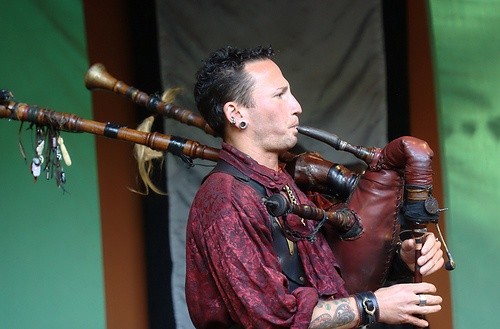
[419,294,427,307]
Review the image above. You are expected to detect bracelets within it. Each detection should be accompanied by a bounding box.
[356,291,381,328]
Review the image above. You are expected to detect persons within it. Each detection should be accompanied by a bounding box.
[183,46,443,328]
